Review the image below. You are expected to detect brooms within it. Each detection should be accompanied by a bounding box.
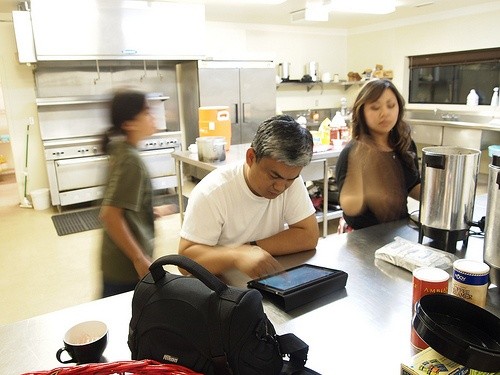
[19,125,33,209]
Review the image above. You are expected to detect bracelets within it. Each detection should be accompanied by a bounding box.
[250,241,257,245]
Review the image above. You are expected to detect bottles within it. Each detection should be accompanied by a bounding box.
[490,87,499,107]
[466,89,479,105]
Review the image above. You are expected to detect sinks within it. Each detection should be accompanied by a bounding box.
[402,118,445,147]
[440,120,500,152]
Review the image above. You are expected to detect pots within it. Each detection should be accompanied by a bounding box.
[483,145,500,289]
[279,62,291,82]
[195,136,227,163]
[419,145,482,250]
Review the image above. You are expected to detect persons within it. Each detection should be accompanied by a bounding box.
[99,89,163,298]
[335,78,421,235]
[177,114,320,281]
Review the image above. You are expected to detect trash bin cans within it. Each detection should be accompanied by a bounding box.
[29,187,51,212]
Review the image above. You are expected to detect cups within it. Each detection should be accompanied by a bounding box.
[411,266,449,355]
[452,258,490,310]
[55,321,108,365]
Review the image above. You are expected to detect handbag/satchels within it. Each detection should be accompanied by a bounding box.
[126,255,310,375]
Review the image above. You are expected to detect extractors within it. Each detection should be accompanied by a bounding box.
[36,92,170,110]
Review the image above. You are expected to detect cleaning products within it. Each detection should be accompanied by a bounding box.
[466,89,479,105]
[490,87,499,109]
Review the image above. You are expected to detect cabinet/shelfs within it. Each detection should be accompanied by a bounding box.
[277,80,369,131]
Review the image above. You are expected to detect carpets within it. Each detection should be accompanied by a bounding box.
[151,192,189,213]
[51,208,104,237]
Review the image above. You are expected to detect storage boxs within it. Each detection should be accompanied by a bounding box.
[329,128,348,140]
[400,346,471,375]
[198,105,232,152]
[310,130,331,145]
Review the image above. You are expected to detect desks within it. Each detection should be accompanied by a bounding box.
[0,194,500,375]
[170,143,345,240]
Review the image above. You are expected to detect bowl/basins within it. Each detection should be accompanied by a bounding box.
[410,293,500,373]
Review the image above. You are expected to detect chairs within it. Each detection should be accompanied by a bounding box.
[300,158,329,239]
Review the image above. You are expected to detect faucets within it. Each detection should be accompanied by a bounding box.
[434,107,449,121]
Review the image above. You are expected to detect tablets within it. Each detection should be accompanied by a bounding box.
[253,264,345,294]
